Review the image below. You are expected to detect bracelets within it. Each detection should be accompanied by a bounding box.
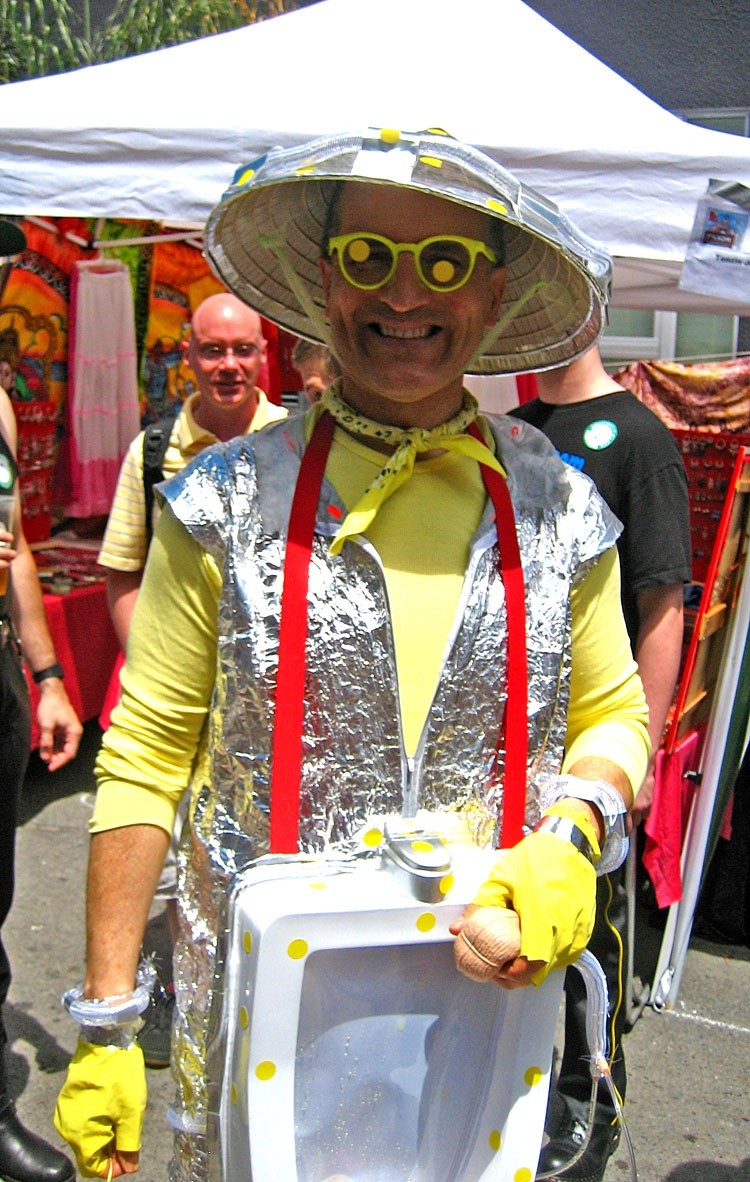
[59,962,157,1029]
[539,771,631,877]
[31,663,64,683]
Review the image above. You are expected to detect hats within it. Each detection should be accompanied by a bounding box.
[201,130,616,376]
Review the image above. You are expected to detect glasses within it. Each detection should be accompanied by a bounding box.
[193,338,266,361]
[325,233,510,294]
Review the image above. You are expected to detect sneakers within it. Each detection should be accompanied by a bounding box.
[135,990,172,1067]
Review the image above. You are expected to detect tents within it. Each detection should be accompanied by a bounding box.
[0,0,750,350]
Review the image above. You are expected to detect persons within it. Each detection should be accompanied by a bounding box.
[96,292,295,1074]
[52,126,656,1182]
[504,332,692,1181]
[0,383,85,1182]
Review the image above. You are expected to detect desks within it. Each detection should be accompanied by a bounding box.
[14,531,122,750]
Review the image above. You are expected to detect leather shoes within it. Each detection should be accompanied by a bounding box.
[537,1115,621,1182]
[0,1084,76,1182]
